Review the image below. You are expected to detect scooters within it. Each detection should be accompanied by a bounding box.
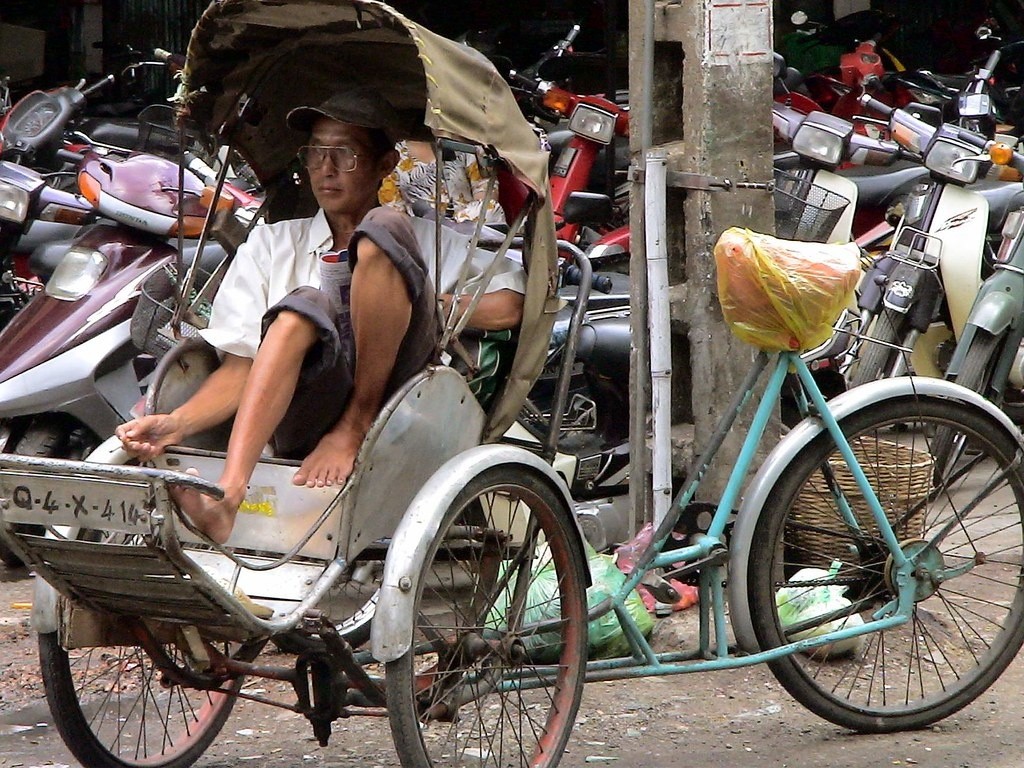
[1,0,1023,574]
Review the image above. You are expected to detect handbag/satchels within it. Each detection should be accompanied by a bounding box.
[481,542,655,658]
[773,556,865,655]
[616,524,703,610]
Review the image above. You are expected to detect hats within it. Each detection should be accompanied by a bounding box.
[287,90,401,147]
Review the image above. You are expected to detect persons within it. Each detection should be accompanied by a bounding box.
[111,89,528,545]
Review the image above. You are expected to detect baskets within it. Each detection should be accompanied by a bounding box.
[783,435,936,591]
[131,264,224,361]
[771,167,851,244]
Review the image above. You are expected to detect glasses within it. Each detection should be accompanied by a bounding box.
[297,143,379,173]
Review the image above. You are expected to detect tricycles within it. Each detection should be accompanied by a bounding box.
[0,0,1024,768]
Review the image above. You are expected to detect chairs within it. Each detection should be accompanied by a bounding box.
[139,301,507,560]
